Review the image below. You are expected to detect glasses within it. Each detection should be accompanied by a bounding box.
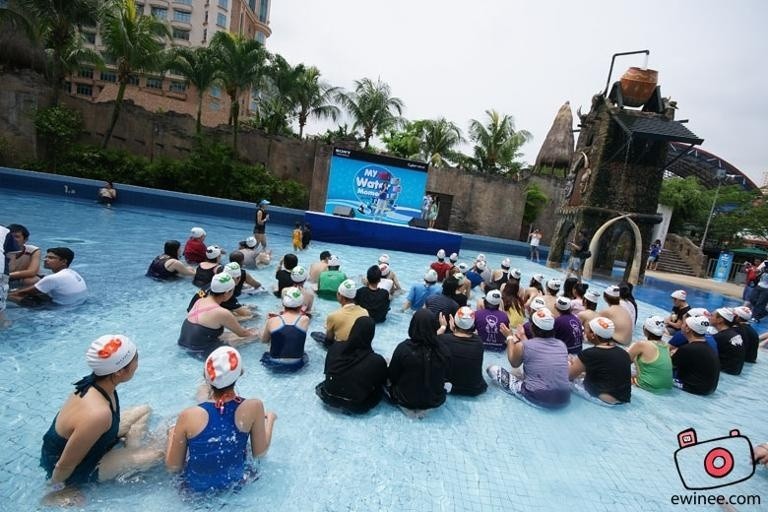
[46,256,59,260]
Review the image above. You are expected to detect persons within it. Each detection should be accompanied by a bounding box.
[40,334,158,503]
[97,181,116,208]
[0,225,22,330]
[753,441,768,470]
[164,345,279,503]
[374,182,387,220]
[145,193,768,415]
[7,246,87,310]
[7,224,41,306]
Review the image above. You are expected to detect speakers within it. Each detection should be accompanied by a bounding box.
[408,217,429,229]
[333,206,355,217]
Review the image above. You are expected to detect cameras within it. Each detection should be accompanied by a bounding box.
[535,230,538,232]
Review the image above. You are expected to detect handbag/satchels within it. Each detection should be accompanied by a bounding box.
[581,251,592,260]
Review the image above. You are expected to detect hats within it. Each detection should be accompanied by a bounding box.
[477,260,486,271]
[455,273,465,286]
[424,269,438,282]
[534,273,545,283]
[328,254,341,267]
[584,289,600,303]
[476,254,486,261]
[671,289,687,300]
[502,257,511,268]
[457,260,469,272]
[687,308,711,320]
[530,297,546,310]
[511,268,521,278]
[548,277,561,290]
[87,334,137,375]
[206,244,221,259]
[190,226,206,238]
[205,346,242,390]
[604,285,620,297]
[437,249,445,259]
[716,307,733,322]
[686,316,709,334]
[380,264,391,276]
[338,279,357,299]
[282,286,304,307]
[291,265,308,283]
[379,254,391,264]
[532,308,555,330]
[556,296,571,310]
[644,315,665,336]
[224,262,242,278]
[211,273,236,293]
[454,306,476,330]
[590,317,615,339]
[450,252,458,261]
[486,289,502,305]
[246,236,257,248]
[734,306,752,321]
[260,200,271,205]
